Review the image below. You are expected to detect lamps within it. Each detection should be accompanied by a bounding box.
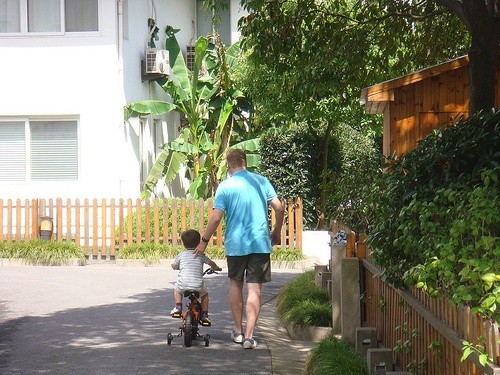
[40,216,53,231]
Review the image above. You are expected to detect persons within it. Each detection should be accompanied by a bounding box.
[193,149,284,349]
[170,229,219,324]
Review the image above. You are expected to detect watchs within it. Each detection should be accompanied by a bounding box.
[202,236,209,242]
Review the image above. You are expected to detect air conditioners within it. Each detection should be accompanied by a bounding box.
[146,48,170,75]
[186,45,196,71]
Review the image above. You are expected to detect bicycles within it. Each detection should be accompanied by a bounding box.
[167,267,222,347]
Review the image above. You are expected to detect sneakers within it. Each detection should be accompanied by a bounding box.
[201,314,211,324]
[231,330,244,343]
[170,307,182,314]
[243,338,257,348]
